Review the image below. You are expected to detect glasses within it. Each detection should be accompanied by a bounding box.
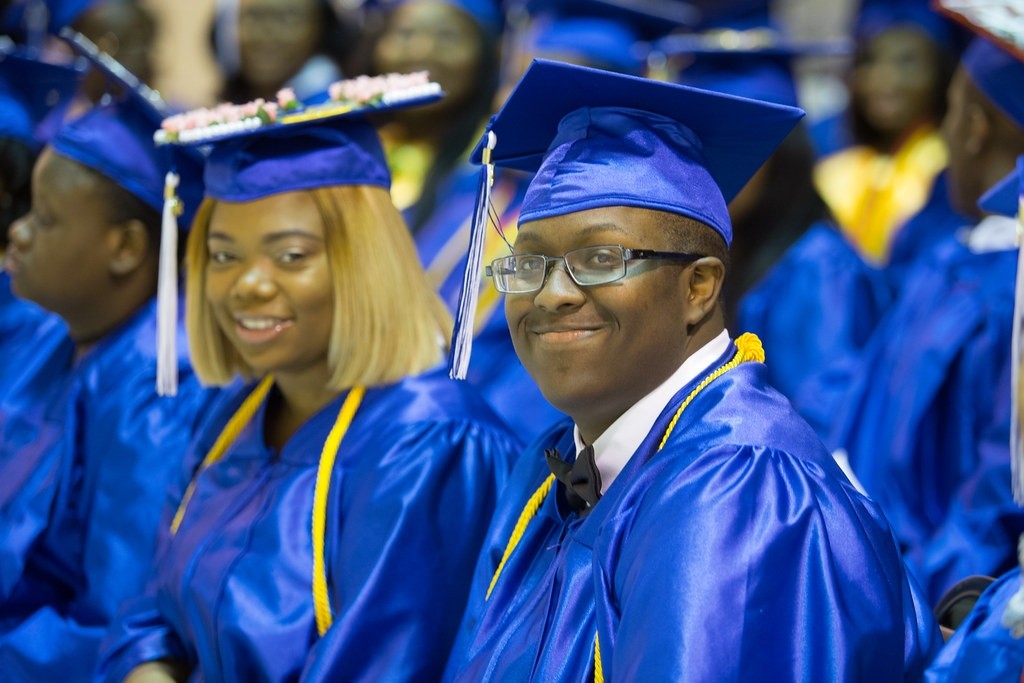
[486,243,702,294]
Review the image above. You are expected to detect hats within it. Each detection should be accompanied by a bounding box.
[378,0,1024,129]
[1,0,94,151]
[154,71,447,398]
[448,56,806,379]
[51,28,210,225]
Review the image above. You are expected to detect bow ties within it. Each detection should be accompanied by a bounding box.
[544,443,602,509]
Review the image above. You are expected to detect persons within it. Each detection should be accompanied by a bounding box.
[0,0,1024,683]
